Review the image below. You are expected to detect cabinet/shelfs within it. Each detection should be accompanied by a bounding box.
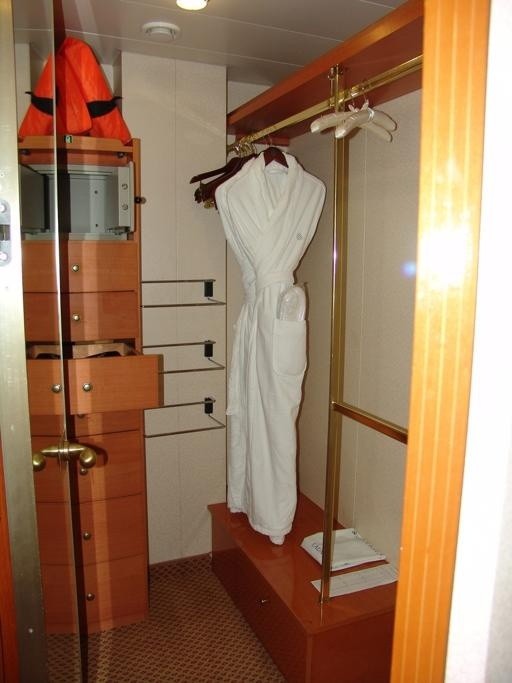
[58,36,164,639]
[19,54,82,632]
[122,52,226,564]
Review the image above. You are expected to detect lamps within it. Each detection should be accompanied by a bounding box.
[139,20,182,44]
[176,0,209,11]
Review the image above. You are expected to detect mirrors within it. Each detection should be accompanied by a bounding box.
[0,0,85,682]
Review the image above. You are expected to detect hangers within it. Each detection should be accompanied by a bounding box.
[335,78,395,138]
[311,87,395,145]
[191,129,291,209]
